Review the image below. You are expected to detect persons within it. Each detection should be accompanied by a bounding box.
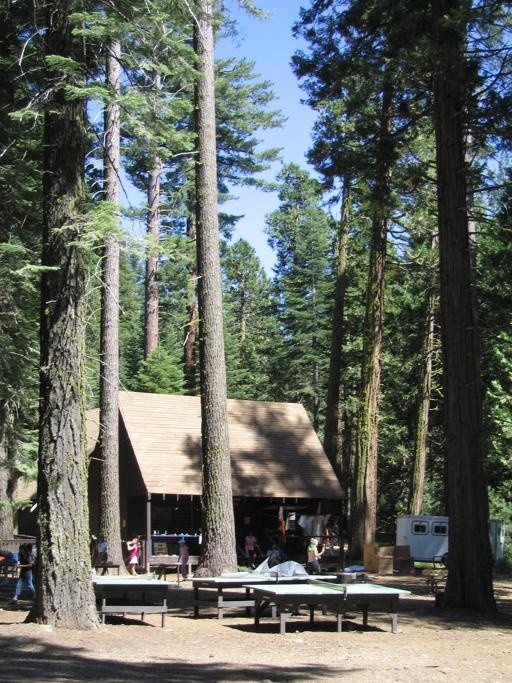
[7,542,37,603]
[305,537,326,574]
[91,534,98,570]
[244,529,258,569]
[175,538,189,579]
[265,543,281,568]
[125,536,142,576]
[92,532,110,577]
